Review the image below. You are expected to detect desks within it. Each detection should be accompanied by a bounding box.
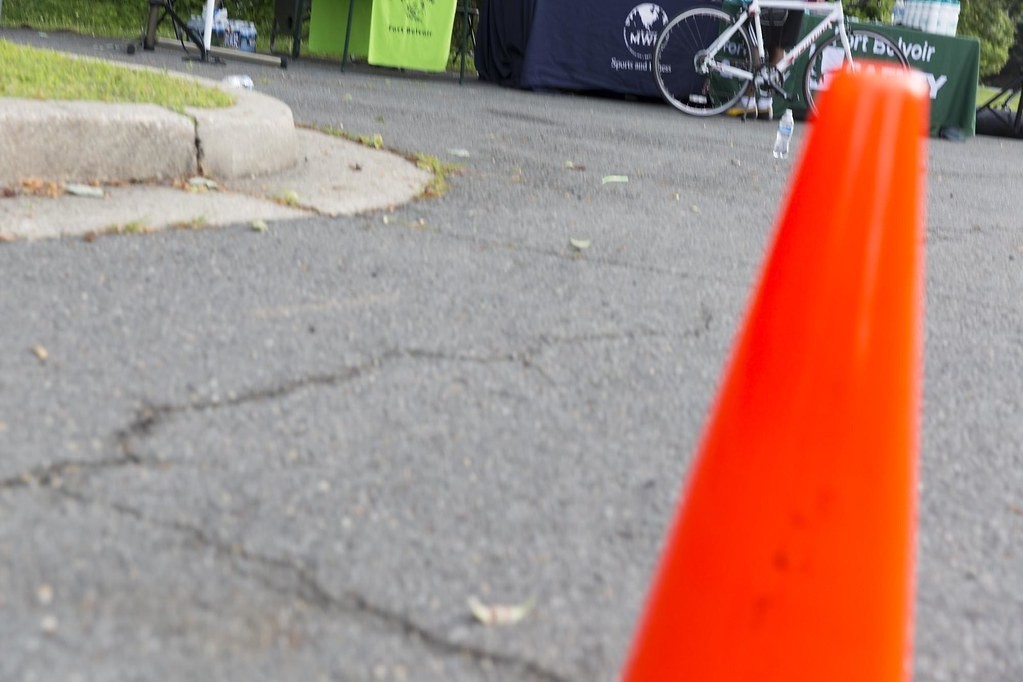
[269,0,980,142]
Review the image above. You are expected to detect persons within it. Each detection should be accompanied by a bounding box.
[726,0,804,121]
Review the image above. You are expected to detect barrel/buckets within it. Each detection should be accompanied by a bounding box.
[186,5,257,54]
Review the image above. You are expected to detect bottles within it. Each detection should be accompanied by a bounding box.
[772,109,795,159]
[895,0,961,38]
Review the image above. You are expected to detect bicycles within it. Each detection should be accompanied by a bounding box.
[652,0,915,123]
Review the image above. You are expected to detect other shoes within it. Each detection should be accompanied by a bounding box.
[741,96,772,111]
